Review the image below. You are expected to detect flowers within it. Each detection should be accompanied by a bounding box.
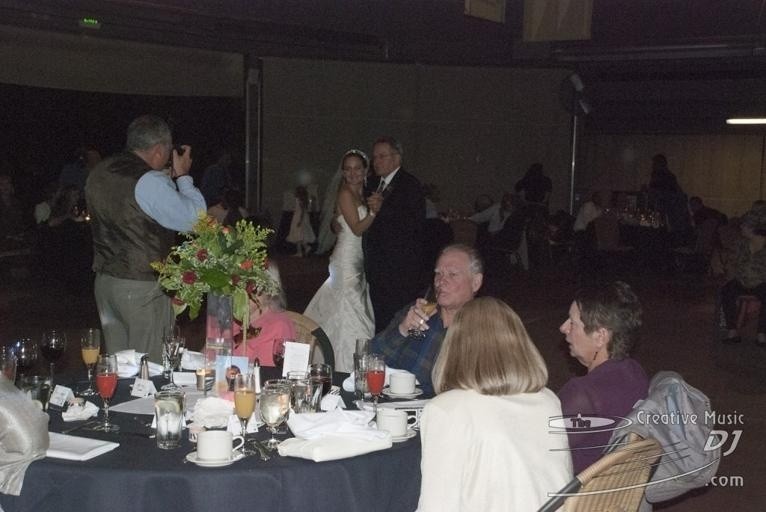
[139,205,286,342]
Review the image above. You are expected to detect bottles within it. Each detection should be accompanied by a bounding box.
[251,357,260,393]
[138,358,148,382]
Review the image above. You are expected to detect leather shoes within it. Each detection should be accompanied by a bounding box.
[720,332,765,345]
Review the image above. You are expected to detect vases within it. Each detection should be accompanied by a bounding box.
[204,290,234,395]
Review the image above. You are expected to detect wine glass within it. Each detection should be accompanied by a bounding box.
[260,363,331,452]
[40,330,65,391]
[233,373,256,456]
[81,328,99,396]
[408,286,441,341]
[350,338,386,409]
[93,352,120,433]
[158,322,184,391]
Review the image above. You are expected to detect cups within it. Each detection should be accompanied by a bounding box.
[378,408,417,434]
[195,430,244,460]
[389,372,418,394]
[194,366,217,391]
[153,390,184,450]
[0,339,52,412]
[273,338,294,372]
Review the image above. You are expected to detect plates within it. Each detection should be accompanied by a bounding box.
[186,452,242,467]
[393,430,417,442]
[383,389,423,400]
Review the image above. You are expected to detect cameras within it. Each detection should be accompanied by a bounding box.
[168,143,193,168]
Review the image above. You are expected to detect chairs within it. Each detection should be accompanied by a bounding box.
[231,309,335,374]
[422,204,766,339]
[532,438,664,511]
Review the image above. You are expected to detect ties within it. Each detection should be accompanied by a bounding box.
[377,181,385,193]
